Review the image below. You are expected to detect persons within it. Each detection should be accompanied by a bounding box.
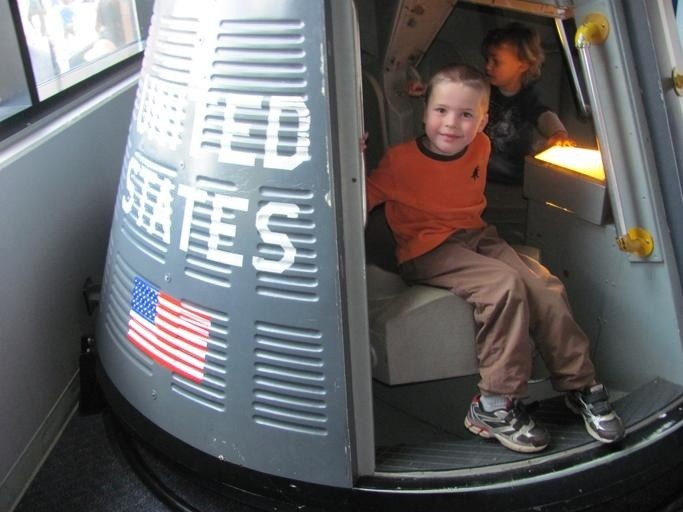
[359,62,626,448]
[405,18,579,185]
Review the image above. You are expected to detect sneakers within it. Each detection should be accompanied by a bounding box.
[464,394,550,454]
[563,385,625,444]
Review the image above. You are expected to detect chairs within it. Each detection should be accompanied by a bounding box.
[367,67,530,227]
